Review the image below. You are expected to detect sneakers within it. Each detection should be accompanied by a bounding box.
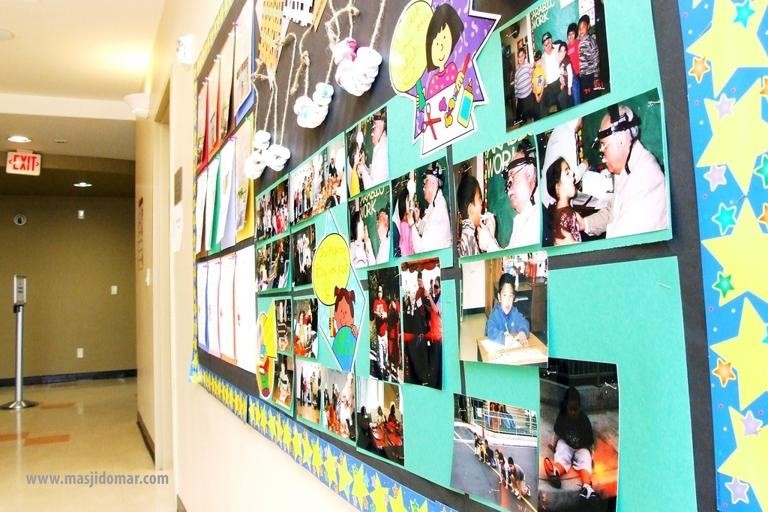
[543,458,560,490]
[579,483,602,503]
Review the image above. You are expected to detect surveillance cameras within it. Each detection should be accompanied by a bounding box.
[13,214,26,226]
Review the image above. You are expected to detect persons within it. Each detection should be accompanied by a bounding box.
[543,386,601,506]
[546,157,581,245]
[456,175,484,256]
[487,273,531,348]
[542,117,590,247]
[554,105,667,247]
[256,112,451,465]
[476,138,538,253]
[513,14,606,126]
[472,434,532,502]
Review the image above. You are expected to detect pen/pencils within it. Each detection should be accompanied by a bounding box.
[504,323,509,332]
